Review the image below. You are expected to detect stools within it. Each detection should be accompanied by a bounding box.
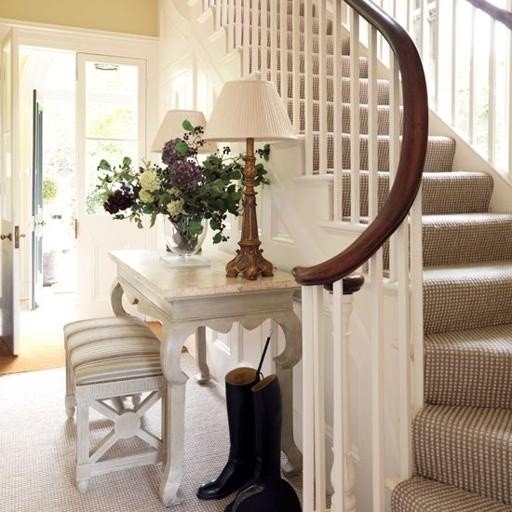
[64,315,192,495]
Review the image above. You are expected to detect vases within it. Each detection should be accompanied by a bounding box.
[164,211,207,257]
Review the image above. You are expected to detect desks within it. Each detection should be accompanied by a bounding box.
[107,248,305,506]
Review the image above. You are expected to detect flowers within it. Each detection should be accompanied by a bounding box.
[85,122,273,240]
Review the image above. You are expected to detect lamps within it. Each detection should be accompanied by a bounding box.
[203,78,302,280]
[152,109,219,255]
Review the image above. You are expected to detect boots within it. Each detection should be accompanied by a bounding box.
[225,375,281,511]
[198,368,262,498]
